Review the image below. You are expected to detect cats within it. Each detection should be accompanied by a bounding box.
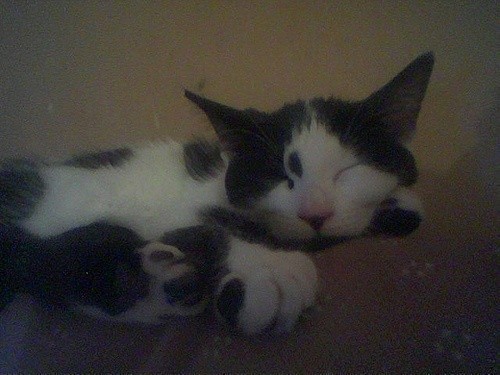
[0,49,436,339]
